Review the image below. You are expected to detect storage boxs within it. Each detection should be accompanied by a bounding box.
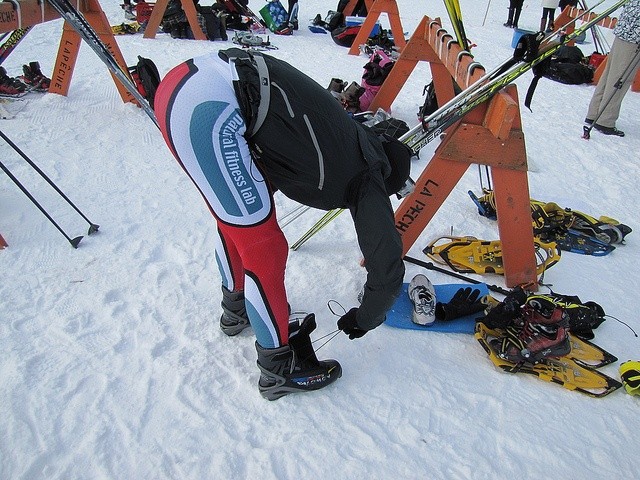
[512,28,535,48]
[346,15,379,40]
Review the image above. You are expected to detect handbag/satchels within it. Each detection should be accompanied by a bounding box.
[359,50,396,116]
[258,0,289,32]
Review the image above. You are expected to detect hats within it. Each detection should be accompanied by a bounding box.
[381,138,415,196]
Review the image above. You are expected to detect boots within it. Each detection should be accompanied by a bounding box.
[512,8,522,28]
[220,285,291,336]
[255,312,342,401]
[503,7,514,28]
[547,19,554,29]
[541,18,547,32]
[327,77,365,113]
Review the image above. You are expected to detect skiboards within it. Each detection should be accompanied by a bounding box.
[290,1,626,251]
[445,0,470,53]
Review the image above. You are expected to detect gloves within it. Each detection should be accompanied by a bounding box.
[435,287,488,321]
[474,285,527,329]
[337,308,368,340]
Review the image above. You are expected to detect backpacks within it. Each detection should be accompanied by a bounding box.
[331,25,362,47]
[417,77,462,134]
[525,46,595,113]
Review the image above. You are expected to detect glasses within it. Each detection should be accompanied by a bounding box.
[393,172,415,200]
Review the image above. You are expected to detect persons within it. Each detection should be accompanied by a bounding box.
[584,0,640,136]
[123,0,145,21]
[558,0,579,12]
[504,0,524,29]
[153,47,411,402]
[540,0,560,31]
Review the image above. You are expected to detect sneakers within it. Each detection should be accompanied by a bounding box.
[23,62,51,92]
[522,294,594,341]
[593,122,624,136]
[0,66,27,94]
[125,10,137,20]
[585,118,594,124]
[531,200,575,242]
[559,207,632,245]
[499,323,571,363]
[618,360,640,396]
[407,274,437,326]
[511,295,569,332]
